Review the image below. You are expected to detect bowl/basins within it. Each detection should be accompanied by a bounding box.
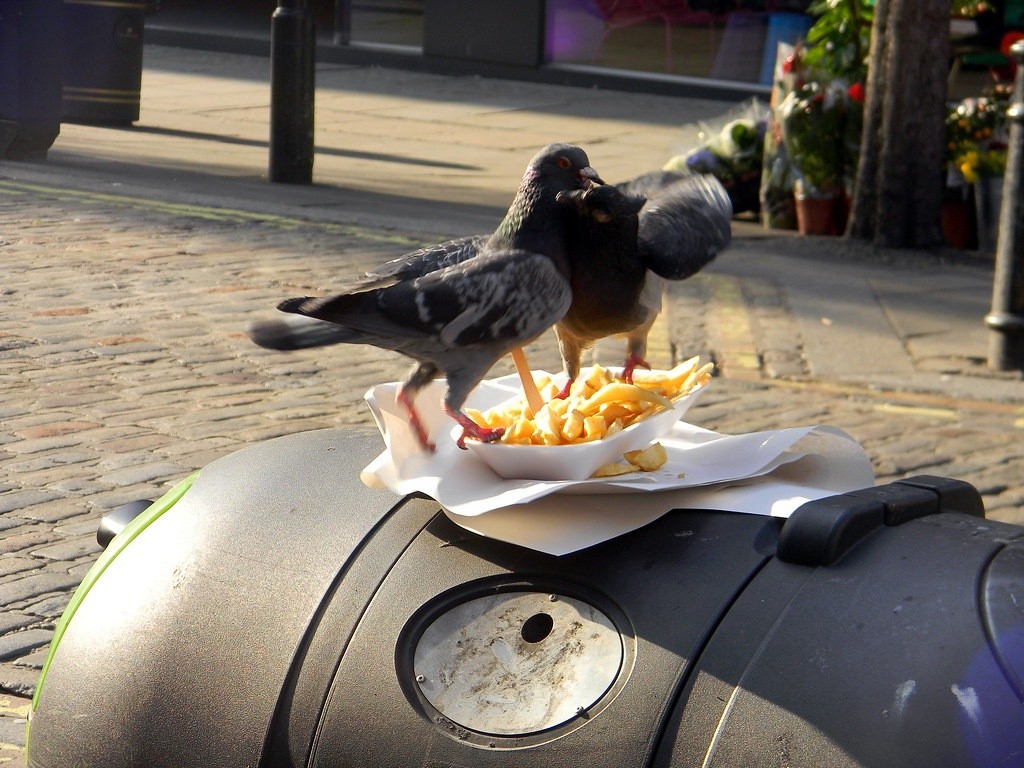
[448,364,713,483]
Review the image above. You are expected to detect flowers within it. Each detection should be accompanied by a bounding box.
[769,0,1024,188]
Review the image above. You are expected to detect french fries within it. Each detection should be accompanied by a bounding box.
[464,355,700,477]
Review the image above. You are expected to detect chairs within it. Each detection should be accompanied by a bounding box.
[591,0,717,75]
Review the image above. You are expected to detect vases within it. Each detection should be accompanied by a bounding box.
[941,206,976,247]
[796,185,836,235]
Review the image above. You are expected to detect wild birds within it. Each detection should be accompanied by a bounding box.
[276,144,665,454]
[344,166,731,403]
